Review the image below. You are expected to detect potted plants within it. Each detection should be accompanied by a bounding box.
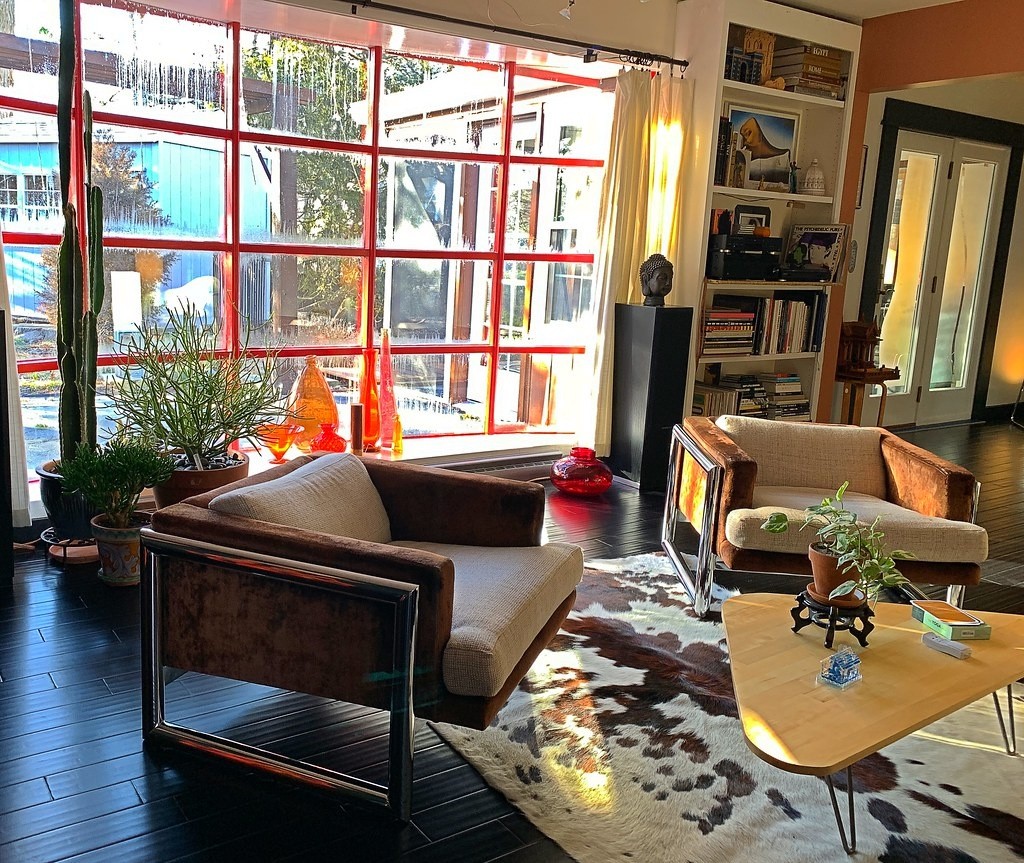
[759,481,918,611]
[36,1,114,539]
[85,290,315,509]
[53,440,179,586]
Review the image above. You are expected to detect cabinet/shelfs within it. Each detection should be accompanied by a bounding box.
[685,0,863,419]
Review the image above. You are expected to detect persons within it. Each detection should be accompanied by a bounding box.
[703,290,827,355]
[788,161,801,194]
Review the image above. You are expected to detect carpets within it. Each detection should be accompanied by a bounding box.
[428,554,1024,862]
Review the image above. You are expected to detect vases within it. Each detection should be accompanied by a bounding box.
[286,327,395,452]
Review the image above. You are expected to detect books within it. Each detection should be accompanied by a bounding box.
[692,362,811,422]
[714,115,751,189]
[771,45,842,100]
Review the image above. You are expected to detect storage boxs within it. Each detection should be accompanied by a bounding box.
[912,604,991,641]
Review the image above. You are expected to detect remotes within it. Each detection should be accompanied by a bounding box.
[921,632,972,660]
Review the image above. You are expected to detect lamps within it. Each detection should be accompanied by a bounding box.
[559,0,576,20]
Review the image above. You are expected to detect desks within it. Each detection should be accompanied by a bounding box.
[721,593,1024,855]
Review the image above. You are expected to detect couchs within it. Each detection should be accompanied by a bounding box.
[661,414,982,618]
[141,453,583,824]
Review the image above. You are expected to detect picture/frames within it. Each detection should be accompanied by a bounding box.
[785,222,848,283]
[724,99,801,189]
